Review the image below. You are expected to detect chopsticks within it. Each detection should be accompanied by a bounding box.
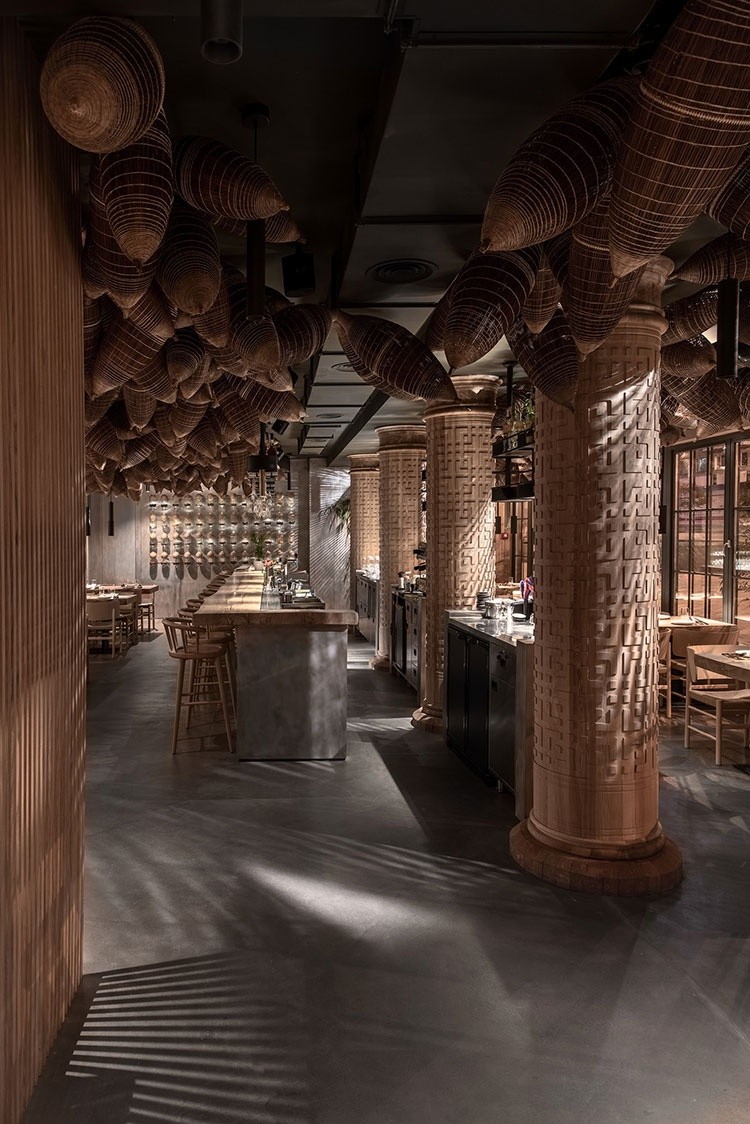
[694,617,710,626]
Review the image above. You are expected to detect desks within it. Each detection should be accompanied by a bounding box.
[93,584,159,631]
[659,615,738,702]
[86,594,137,649]
[694,652,750,685]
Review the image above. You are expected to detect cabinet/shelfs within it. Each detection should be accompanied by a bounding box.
[441,621,519,796]
[390,598,422,705]
[356,578,378,648]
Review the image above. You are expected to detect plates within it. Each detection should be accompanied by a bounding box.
[671,620,696,625]
[511,612,526,619]
[659,614,670,619]
[736,649,750,658]
[98,595,112,599]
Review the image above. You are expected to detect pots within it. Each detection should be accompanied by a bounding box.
[484,599,515,619]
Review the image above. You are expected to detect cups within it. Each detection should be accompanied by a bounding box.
[91,576,97,586]
[508,577,514,584]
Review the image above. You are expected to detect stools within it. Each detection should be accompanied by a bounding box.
[162,574,235,755]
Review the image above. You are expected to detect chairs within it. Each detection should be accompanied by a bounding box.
[85,584,153,663]
[659,627,750,767]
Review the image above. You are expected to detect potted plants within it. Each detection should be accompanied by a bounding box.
[253,536,264,571]
